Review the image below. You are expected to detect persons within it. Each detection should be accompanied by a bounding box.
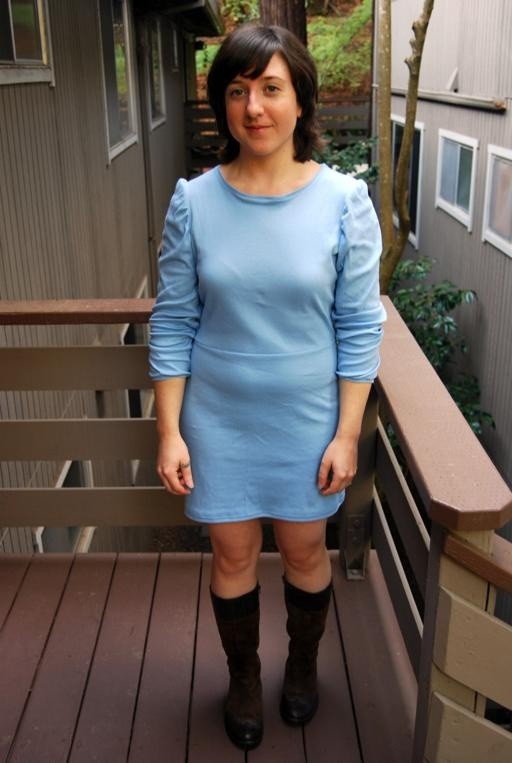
[147,19,388,748]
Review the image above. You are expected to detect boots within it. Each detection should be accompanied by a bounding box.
[210,581,264,751]
[281,572,333,728]
